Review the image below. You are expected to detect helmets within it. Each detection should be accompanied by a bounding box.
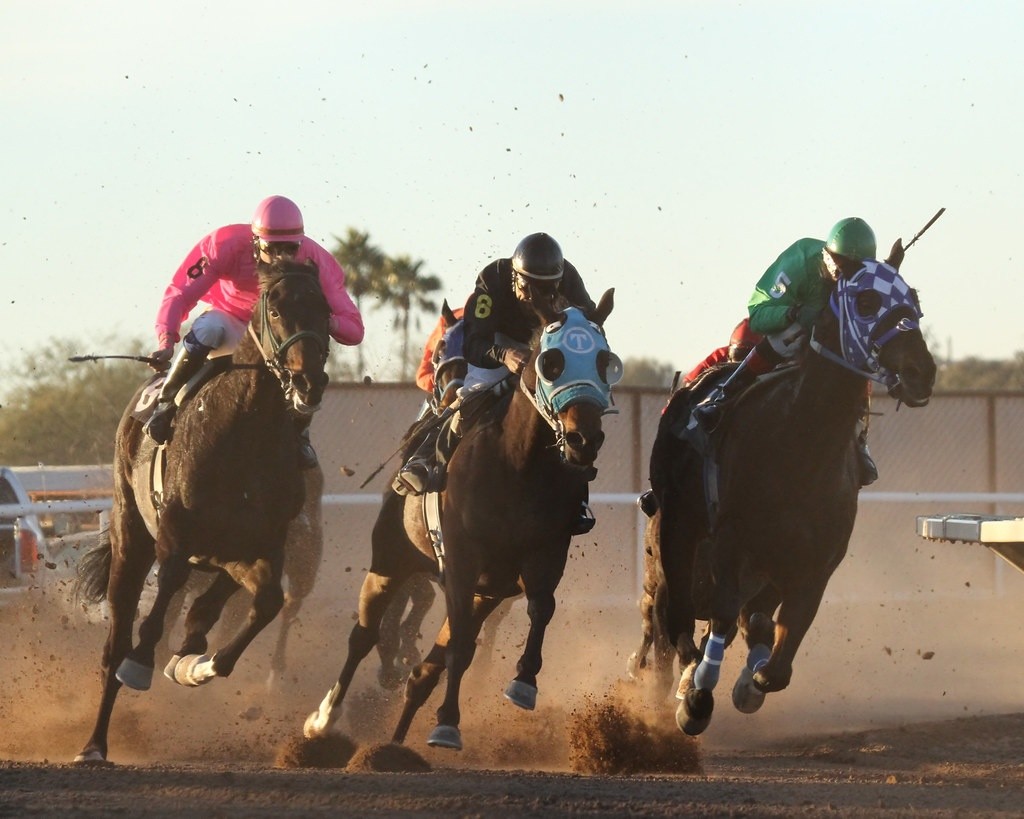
[826,218,876,260]
[513,233,564,279]
[251,196,305,241]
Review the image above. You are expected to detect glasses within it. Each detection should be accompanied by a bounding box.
[259,243,299,256]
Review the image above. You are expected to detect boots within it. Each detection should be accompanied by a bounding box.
[392,406,457,496]
[693,345,776,433]
[150,331,211,445]
[301,438,318,468]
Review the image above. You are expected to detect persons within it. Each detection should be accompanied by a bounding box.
[636,216,877,516]
[141,195,366,466]
[394,231,609,495]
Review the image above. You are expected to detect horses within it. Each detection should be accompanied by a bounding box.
[301,280,626,752]
[66,247,334,765]
[624,236,939,739]
